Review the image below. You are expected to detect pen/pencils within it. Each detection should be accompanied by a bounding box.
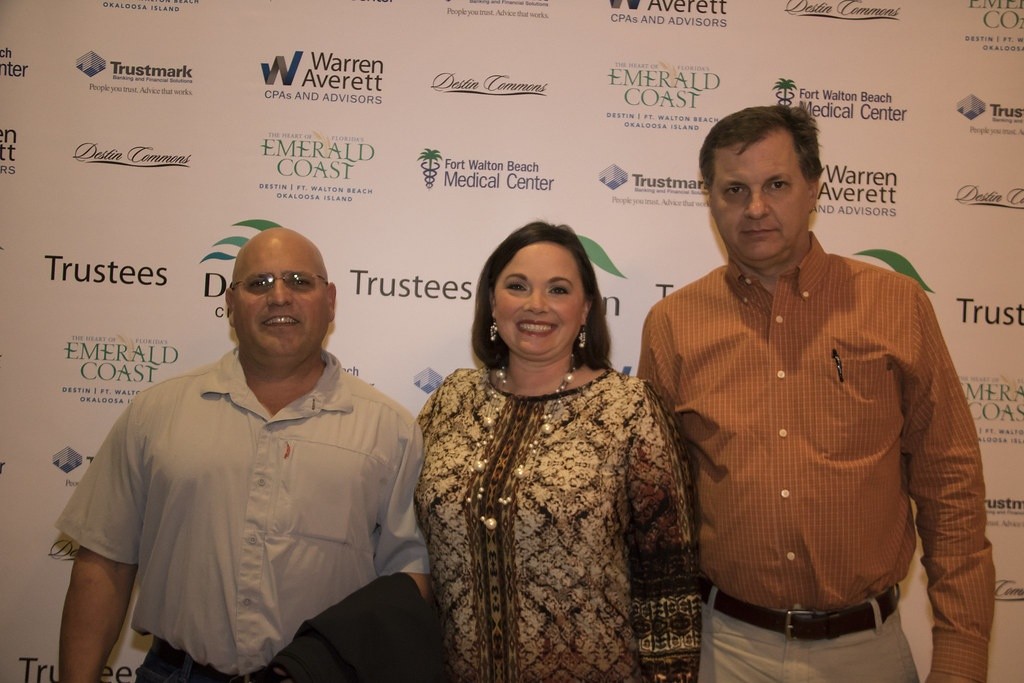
[830,349,846,384]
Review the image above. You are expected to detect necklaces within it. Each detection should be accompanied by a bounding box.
[465,353,577,528]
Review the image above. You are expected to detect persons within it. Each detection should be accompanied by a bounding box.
[413,221,704,683]
[55,227,431,683]
[636,106,997,683]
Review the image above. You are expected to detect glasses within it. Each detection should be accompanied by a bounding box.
[230,272,328,295]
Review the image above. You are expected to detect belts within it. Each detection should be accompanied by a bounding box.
[696,574,900,643]
